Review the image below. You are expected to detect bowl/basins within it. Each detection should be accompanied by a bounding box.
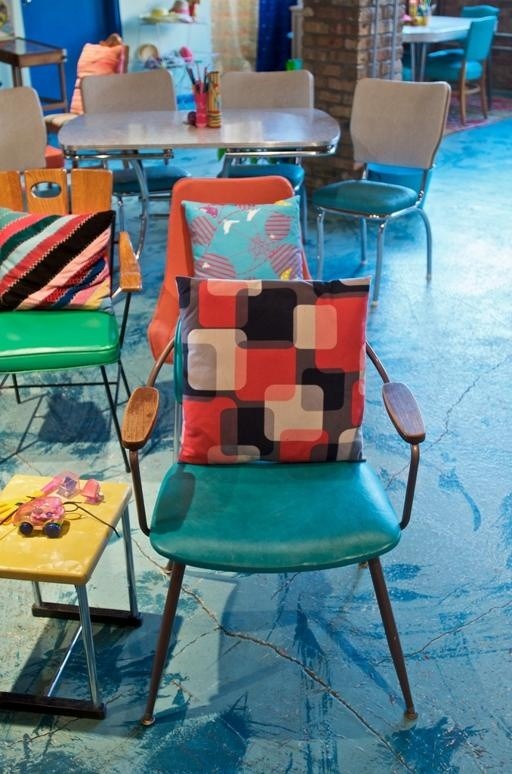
[151,6,168,17]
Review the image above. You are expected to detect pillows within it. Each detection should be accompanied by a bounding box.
[173,276,374,467]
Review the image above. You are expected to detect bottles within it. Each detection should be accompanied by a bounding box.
[193,71,224,129]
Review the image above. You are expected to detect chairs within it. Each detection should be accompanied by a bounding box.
[309,76,451,310]
[118,326,427,733]
[402,5,503,125]
[0,67,341,476]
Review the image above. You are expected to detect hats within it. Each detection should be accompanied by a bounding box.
[141,0,192,23]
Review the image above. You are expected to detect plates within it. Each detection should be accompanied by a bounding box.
[137,42,159,65]
[140,13,179,22]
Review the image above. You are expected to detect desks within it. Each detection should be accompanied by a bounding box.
[0,474,143,721]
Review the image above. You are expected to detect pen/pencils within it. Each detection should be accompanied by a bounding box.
[185,63,208,94]
